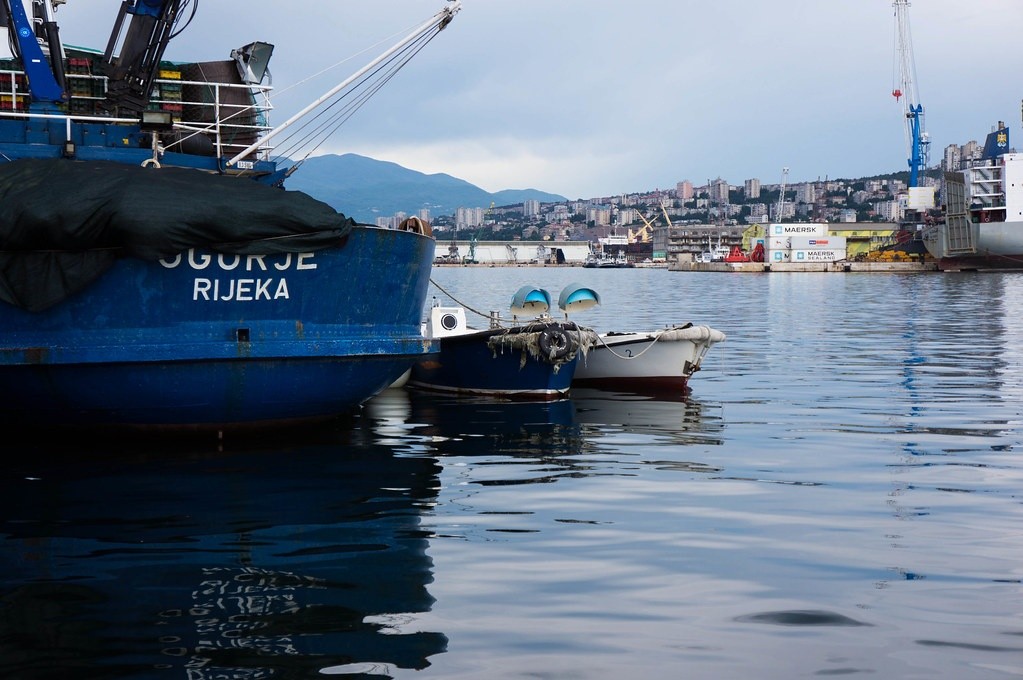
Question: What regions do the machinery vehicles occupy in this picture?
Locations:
[505,244,518,265]
[462,201,494,264]
[431,215,463,264]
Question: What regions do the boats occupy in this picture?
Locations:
[616,250,635,268]
[700,237,715,262]
[921,121,1023,261]
[582,241,599,268]
[507,284,726,393]
[0,0,465,443]
[711,234,730,260]
[597,240,617,268]
[597,220,654,263]
[408,297,597,405]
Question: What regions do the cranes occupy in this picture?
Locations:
[776,163,789,223]
[891,0,931,187]
